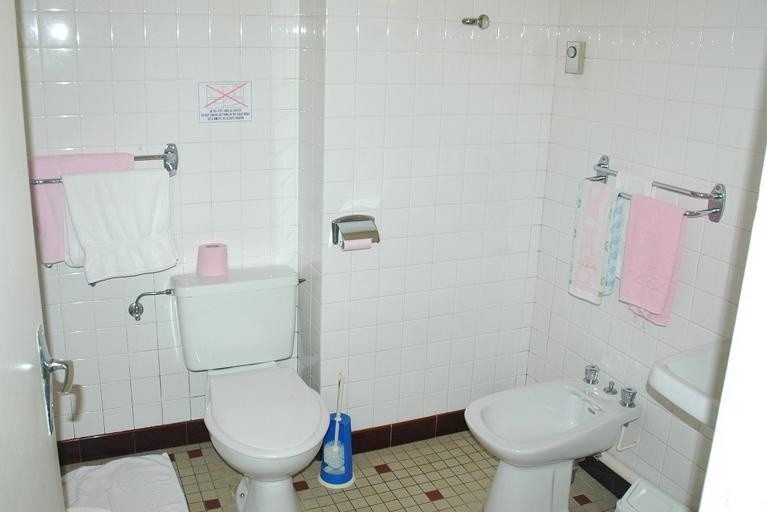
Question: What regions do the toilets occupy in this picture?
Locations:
[170,267,332,511]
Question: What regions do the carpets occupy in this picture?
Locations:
[61,452,191,512]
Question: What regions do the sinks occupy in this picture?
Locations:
[465,379,643,466]
[648,335,732,428]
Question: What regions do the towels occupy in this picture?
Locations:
[27,154,136,265]
[618,195,685,328]
[565,180,618,305]
[63,170,180,285]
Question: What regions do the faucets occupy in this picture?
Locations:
[604,380,618,395]
